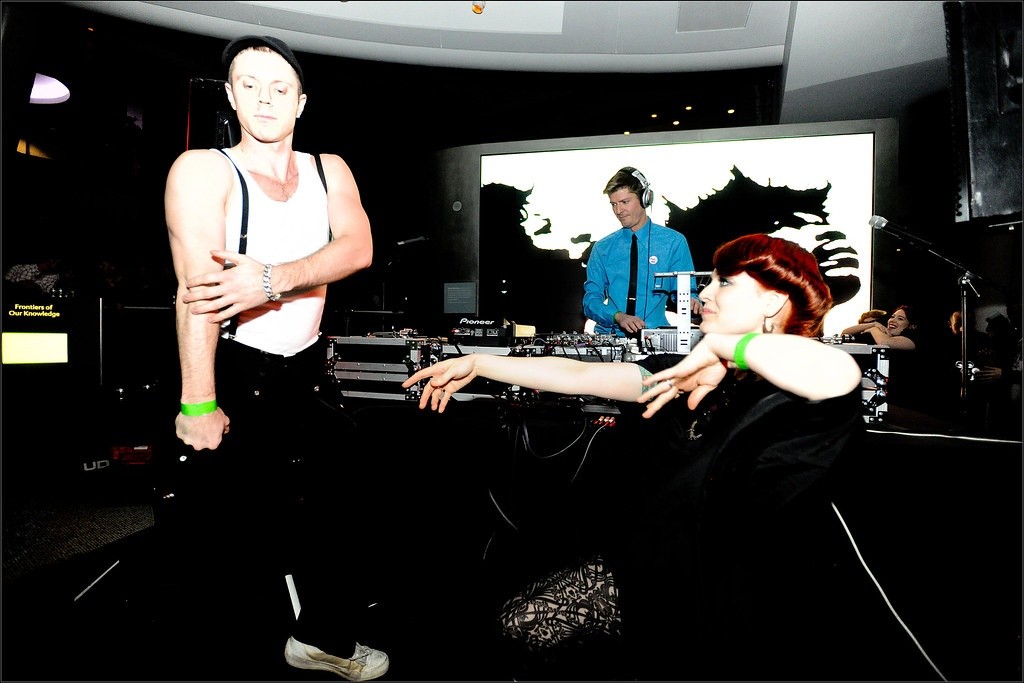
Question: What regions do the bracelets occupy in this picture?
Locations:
[734,332,760,370]
[263,263,282,302]
[613,311,621,321]
[180,399,217,416]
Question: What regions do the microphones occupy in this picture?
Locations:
[868,215,931,249]
[398,236,429,246]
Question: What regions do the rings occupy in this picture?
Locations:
[666,379,672,388]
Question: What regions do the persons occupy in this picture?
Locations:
[164,35,390,682]
[983,365,1022,385]
[402,233,863,651]
[947,310,995,366]
[584,167,703,337]
[842,305,945,419]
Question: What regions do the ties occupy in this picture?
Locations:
[625,234,638,337]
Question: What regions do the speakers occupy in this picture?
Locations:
[943,0,1024,224]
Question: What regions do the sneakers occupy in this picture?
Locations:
[283,634,391,683]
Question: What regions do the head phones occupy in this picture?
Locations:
[619,167,654,209]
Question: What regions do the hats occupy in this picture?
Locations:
[220,34,305,93]
